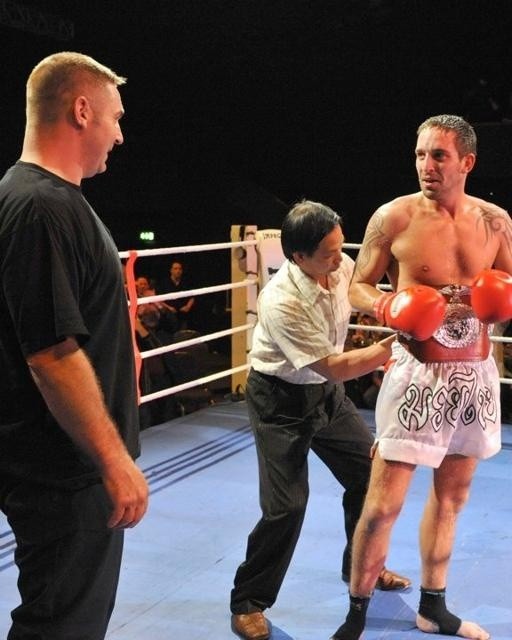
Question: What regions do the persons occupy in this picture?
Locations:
[121,259,195,431]
[0,48,152,640]
[329,113,512,640]
[343,312,395,411]
[227,196,397,640]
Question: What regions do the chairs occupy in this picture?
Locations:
[162,330,217,417]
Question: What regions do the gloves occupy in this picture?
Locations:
[373,284,445,342]
[471,269,512,324]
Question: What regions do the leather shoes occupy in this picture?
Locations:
[232,612,270,640]
[342,567,410,590]
